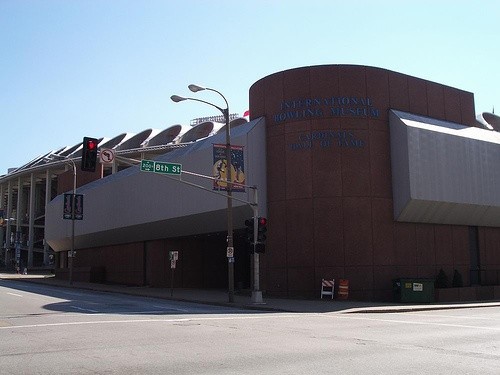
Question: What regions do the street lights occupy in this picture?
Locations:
[42,153,77,285]
[169,83,235,304]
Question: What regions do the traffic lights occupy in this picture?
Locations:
[245,218,253,241]
[80,136,98,172]
[257,216,268,242]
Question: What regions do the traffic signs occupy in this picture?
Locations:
[154,161,182,176]
[141,159,154,172]
